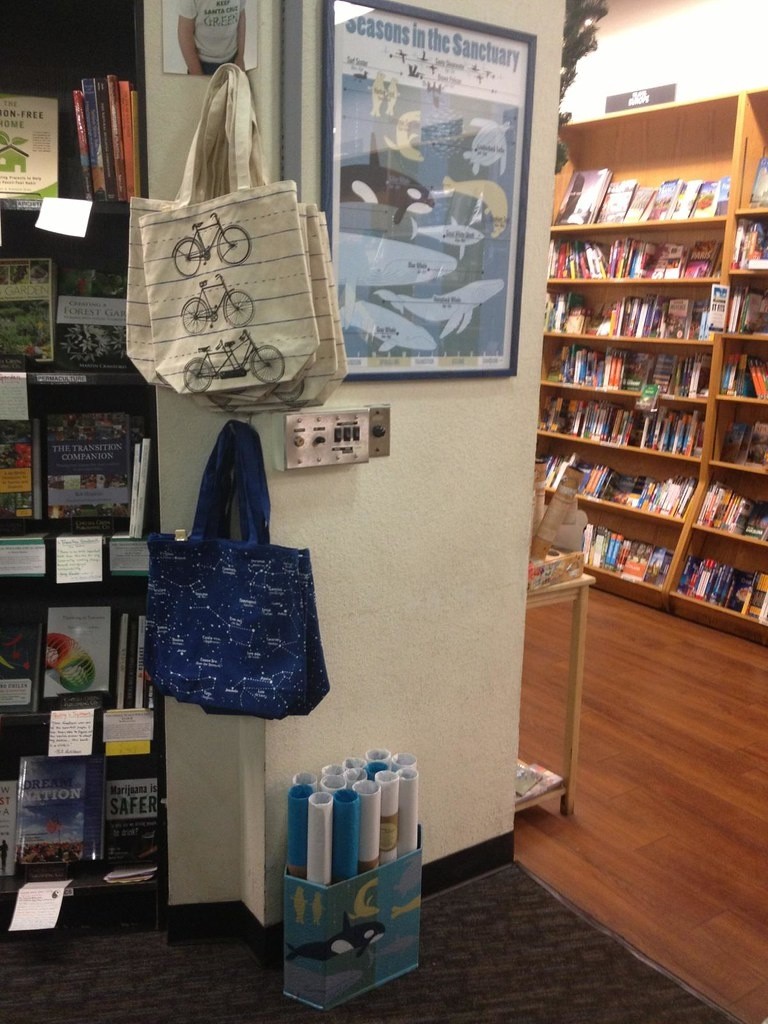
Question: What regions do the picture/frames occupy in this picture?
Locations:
[318,0,538,381]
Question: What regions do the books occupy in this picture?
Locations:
[547,345,710,398]
[552,167,727,225]
[677,555,768,624]
[730,218,768,271]
[541,290,710,345]
[707,286,766,336]
[0,75,168,912]
[538,447,698,519]
[696,479,767,542]
[548,235,723,285]
[745,147,768,209]
[721,419,768,469]
[538,393,703,459]
[578,522,674,590]
[720,353,767,399]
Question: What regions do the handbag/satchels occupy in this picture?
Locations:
[150,420,335,722]
[128,63,347,413]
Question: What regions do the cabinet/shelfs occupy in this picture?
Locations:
[666,87,768,647]
[0,0,171,934]
[532,88,747,615]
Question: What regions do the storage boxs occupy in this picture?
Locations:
[283,823,422,1009]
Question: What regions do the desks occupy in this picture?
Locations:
[514,572,598,818]
